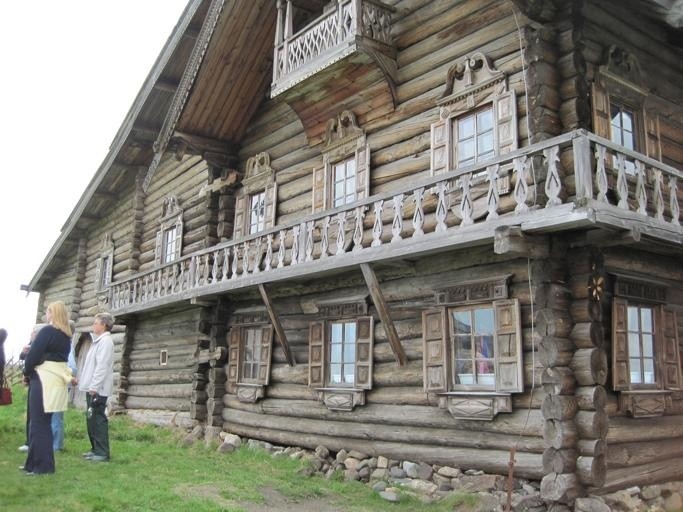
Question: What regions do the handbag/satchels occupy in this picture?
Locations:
[0,378,12,406]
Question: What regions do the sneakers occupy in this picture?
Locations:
[18,445,29,451]
[83,452,110,462]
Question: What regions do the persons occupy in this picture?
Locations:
[18,300,115,476]
[0,328,12,405]
